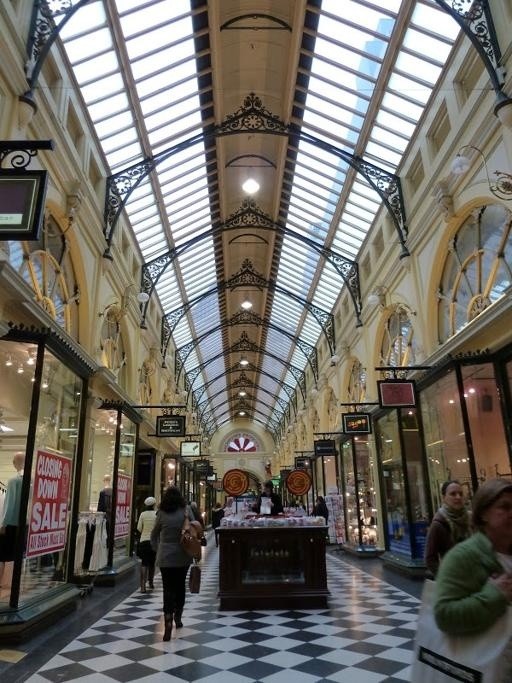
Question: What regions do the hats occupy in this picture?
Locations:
[143,496,156,506]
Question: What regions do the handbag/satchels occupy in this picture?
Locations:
[201,536,206,545]
[189,566,201,593]
[180,504,204,563]
[409,575,512,681]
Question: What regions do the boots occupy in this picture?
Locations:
[140,565,147,592]
[147,567,154,587]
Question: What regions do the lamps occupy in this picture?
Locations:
[295,284,419,417]
[173,390,209,443]
[98,281,150,324]
[448,141,511,201]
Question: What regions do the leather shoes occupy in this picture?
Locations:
[175,621,183,629]
[163,621,172,641]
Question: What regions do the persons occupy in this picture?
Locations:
[212,502,225,546]
[298,499,307,509]
[137,484,204,642]
[1,452,26,594]
[98,474,111,512]
[253,480,283,516]
[432,479,512,635]
[426,480,473,575]
[312,496,328,525]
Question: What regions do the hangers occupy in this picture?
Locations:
[75,510,105,527]
